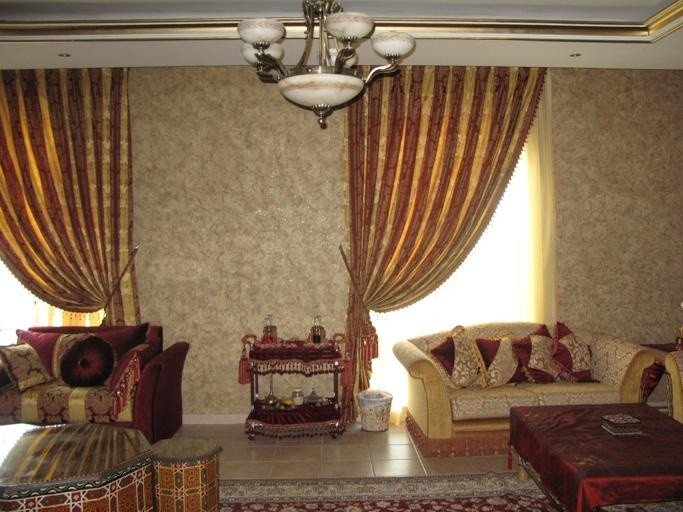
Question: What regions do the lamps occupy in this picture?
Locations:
[236,0,419,125]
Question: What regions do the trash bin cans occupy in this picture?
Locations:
[357,389,393,432]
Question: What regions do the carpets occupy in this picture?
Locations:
[217,476,554,511]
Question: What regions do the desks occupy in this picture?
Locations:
[0,423,223,510]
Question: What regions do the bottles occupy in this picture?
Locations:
[310,317,324,344]
[256,375,329,410]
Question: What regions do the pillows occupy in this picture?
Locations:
[428,323,595,387]
[0,331,152,391]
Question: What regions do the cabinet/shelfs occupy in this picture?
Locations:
[246,338,347,439]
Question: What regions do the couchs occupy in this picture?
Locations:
[392,324,670,439]
[0,325,189,441]
